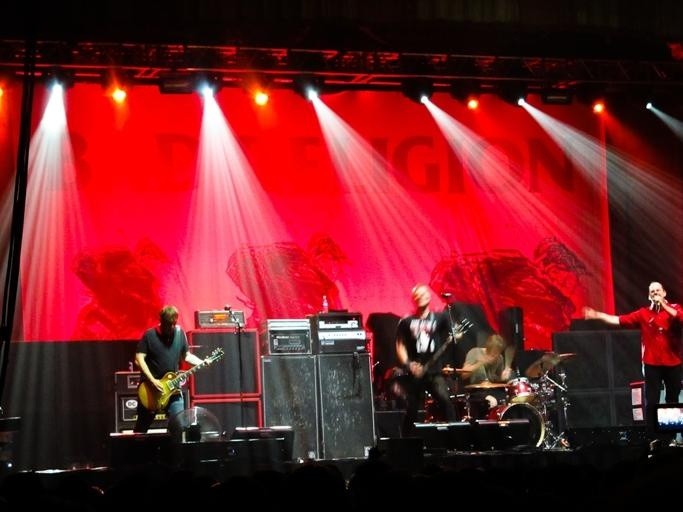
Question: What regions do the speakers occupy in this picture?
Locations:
[261,355,320,460]
[190,398,264,436]
[317,353,377,460]
[234,426,294,459]
[179,329,262,399]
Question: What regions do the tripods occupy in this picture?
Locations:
[541,374,567,450]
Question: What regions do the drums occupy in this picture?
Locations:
[489,403,545,448]
[506,377,535,403]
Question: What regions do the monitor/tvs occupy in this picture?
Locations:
[654,404,683,432]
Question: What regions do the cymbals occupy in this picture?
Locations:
[442,367,472,374]
[526,359,551,379]
[465,380,511,389]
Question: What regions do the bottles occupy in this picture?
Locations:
[675,432,683,447]
[322,295,329,313]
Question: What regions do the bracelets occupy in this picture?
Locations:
[405,360,412,369]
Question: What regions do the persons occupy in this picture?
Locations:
[586,281,683,426]
[133,304,211,442]
[392,284,464,422]
[1,440,683,510]
[462,334,507,417]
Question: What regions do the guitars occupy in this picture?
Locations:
[138,348,224,412]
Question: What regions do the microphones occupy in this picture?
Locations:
[442,293,452,297]
[656,301,661,313]
[224,305,232,310]
